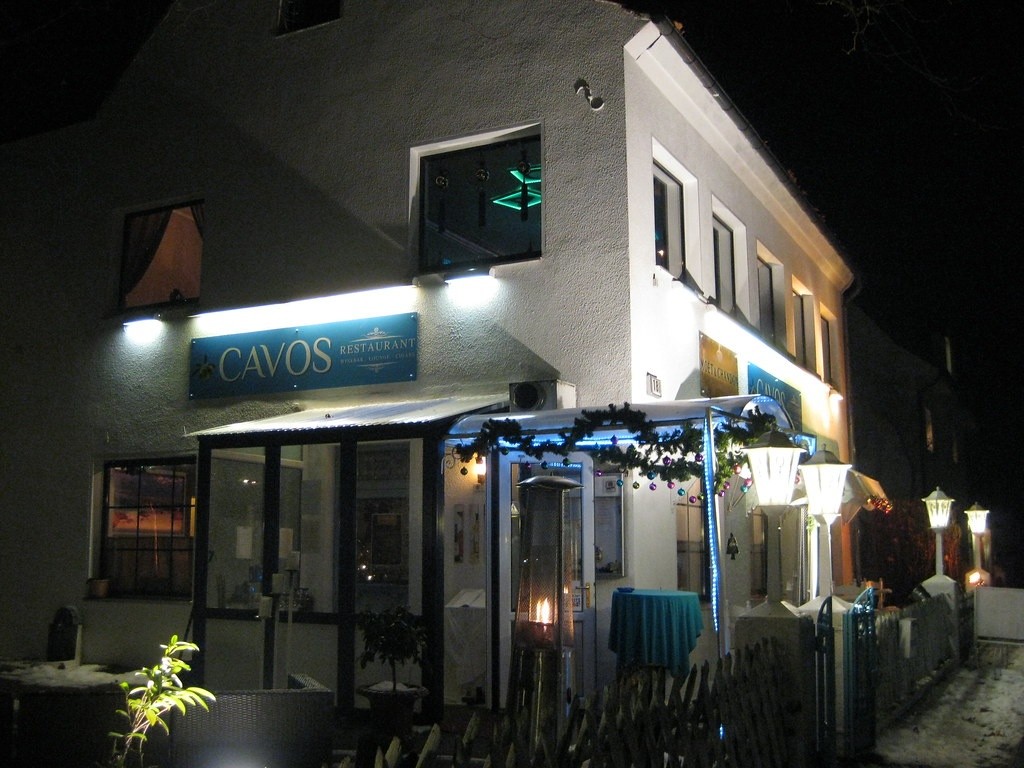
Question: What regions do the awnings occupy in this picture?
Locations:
[731,449,889,528]
[446,392,797,448]
[181,394,512,705]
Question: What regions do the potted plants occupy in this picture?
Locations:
[349,603,438,733]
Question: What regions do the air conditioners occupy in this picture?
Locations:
[507,379,578,415]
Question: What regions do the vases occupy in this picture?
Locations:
[88,577,112,598]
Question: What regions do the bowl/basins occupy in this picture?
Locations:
[139,575,174,601]
[89,580,113,599]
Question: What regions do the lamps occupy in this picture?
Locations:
[726,533,741,561]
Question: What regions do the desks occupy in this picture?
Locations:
[608,587,705,680]
